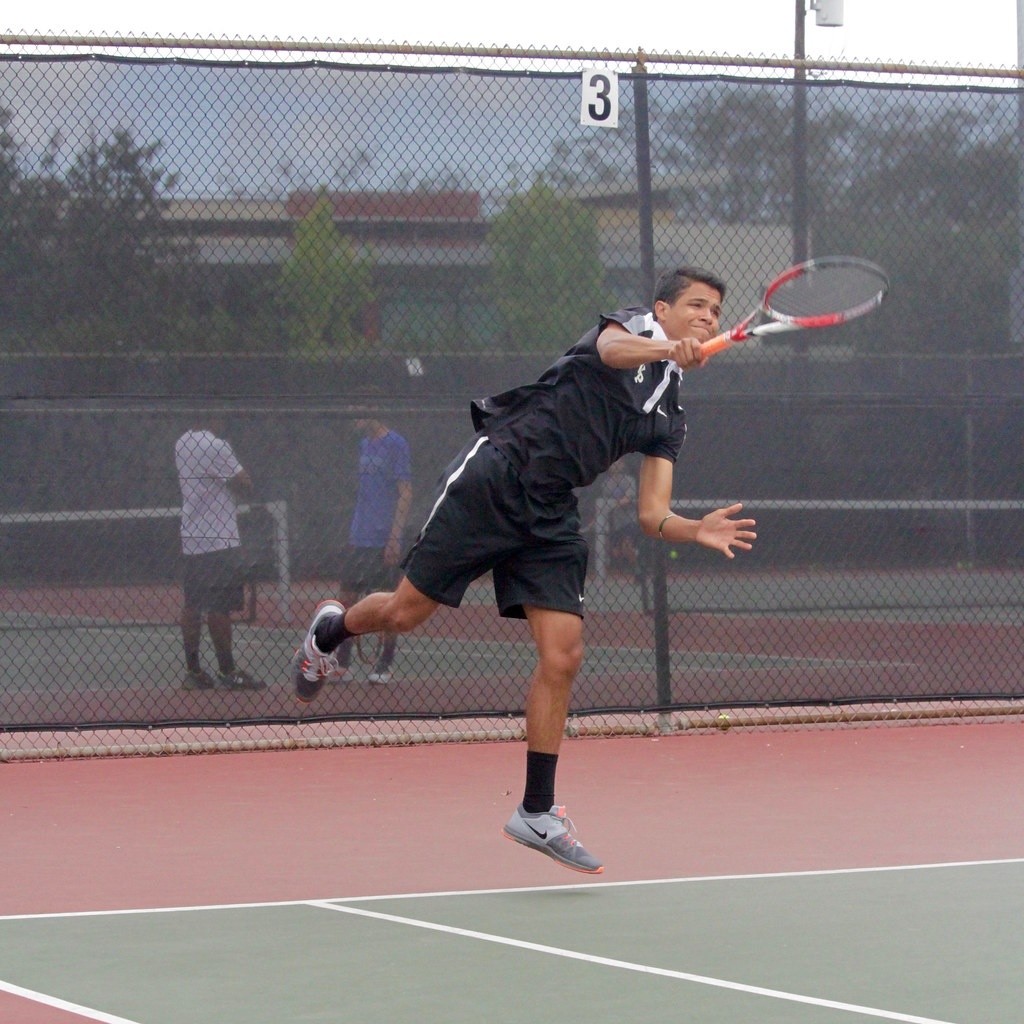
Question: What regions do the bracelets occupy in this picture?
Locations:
[657,513,679,541]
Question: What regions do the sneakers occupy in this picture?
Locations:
[370,662,396,685]
[183,670,215,690]
[229,672,268,689]
[290,600,347,705]
[499,803,605,875]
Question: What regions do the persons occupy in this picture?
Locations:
[174,393,263,692]
[287,265,756,875]
[580,455,646,586]
[329,382,414,683]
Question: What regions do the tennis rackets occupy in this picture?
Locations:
[701,259,892,357]
